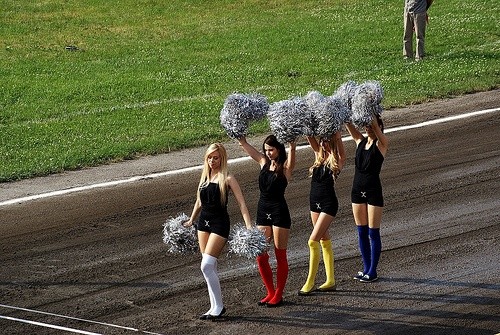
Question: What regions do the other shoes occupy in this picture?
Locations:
[360,275,378,282]
[353,272,364,279]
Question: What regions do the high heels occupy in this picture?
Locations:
[209,306,226,322]
[265,300,283,307]
[316,283,336,292]
[298,286,316,296]
[258,297,269,305]
[201,314,209,319]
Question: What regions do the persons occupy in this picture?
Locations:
[343,112,389,283]
[298,130,346,296]
[235,134,296,308]
[182,143,254,321]
[402,0,433,62]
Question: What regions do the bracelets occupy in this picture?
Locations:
[239,139,246,146]
[289,148,295,151]
